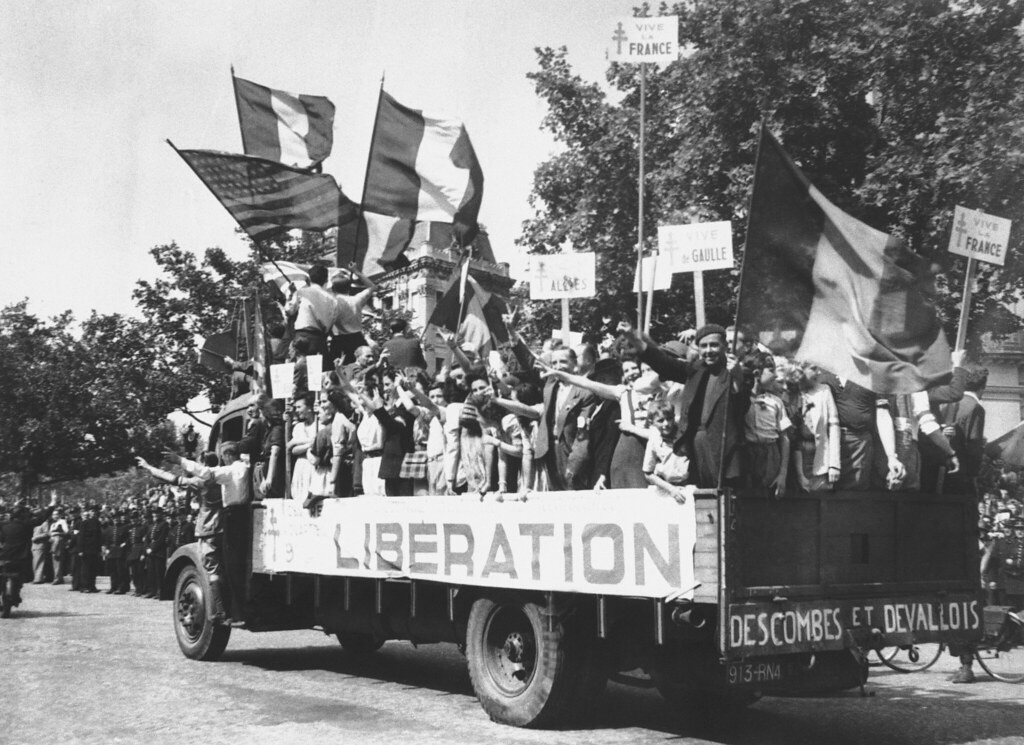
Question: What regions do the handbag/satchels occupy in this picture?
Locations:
[399,452,426,479]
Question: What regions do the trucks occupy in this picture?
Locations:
[160,392,984,728]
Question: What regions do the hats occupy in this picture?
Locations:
[152,507,165,514]
[130,511,139,519]
[586,358,623,381]
[174,508,186,516]
[696,324,725,344]
[68,507,80,514]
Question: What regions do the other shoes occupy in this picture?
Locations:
[80,587,91,593]
[144,592,156,598]
[135,592,144,597]
[115,587,126,594]
[106,588,115,594]
[52,578,63,585]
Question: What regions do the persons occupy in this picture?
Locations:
[47,484,201,598]
[280,267,984,501]
[943,457,1024,683]
[130,356,292,627]
[0,488,58,618]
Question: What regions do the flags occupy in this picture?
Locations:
[180,148,361,241]
[234,78,337,171]
[334,197,415,278]
[261,260,358,301]
[737,123,954,396]
[423,277,511,363]
[363,92,485,230]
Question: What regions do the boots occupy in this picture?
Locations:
[210,580,227,621]
